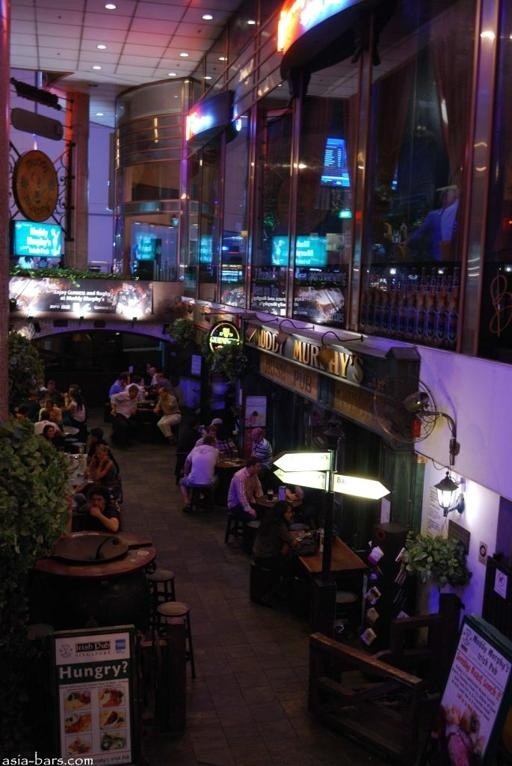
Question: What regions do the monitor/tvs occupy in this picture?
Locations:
[319,136,399,191]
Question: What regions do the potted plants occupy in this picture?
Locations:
[403,530,473,588]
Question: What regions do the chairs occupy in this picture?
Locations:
[16,389,123,531]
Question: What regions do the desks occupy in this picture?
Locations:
[32,532,156,627]
[135,392,162,447]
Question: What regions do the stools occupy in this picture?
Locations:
[170,423,336,611]
[152,600,195,680]
[143,568,177,640]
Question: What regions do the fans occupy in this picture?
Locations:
[373,370,460,465]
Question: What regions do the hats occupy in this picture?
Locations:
[210,418,223,426]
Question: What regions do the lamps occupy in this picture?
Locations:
[317,330,363,371]
[434,471,464,517]
[241,314,278,342]
[276,318,314,345]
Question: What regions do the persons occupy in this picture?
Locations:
[397,184,463,263]
[420,703,452,766]
[18,257,61,270]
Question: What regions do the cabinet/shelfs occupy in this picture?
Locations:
[362,523,421,654]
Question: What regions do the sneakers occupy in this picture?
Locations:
[182,501,193,512]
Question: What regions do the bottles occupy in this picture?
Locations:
[77,446,86,474]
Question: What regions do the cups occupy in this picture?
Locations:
[232,449,239,460]
[267,490,275,500]
